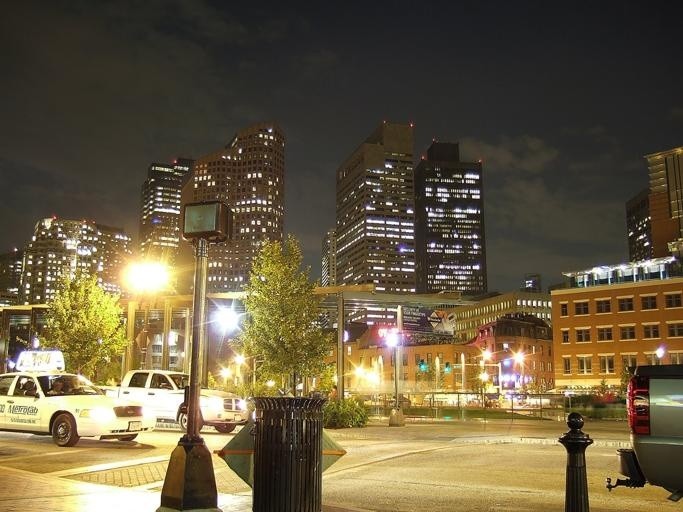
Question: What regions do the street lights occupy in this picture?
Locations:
[654,347,663,366]
[385,333,398,407]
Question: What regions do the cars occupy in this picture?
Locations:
[0,371,157,447]
[363,393,411,409]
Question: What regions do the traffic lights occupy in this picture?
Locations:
[445,361,450,373]
[418,359,424,369]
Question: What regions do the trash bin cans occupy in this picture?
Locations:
[252,398,328,511]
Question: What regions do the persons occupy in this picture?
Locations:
[169,376,179,388]
[326,385,337,400]
[45,378,65,395]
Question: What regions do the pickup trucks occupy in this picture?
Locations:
[606,364,682,503]
[96,369,249,433]
